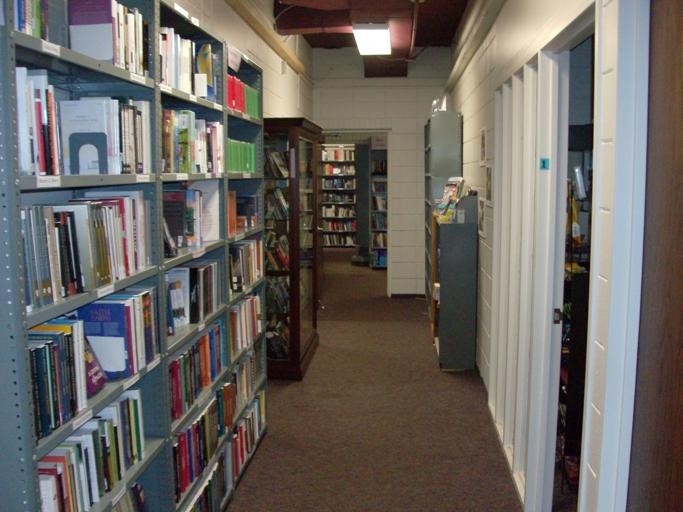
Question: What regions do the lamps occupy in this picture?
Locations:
[351,19,393,57]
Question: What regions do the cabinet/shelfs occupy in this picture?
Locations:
[430,195,482,375]
[263,116,326,382]
[226,47,267,511]
[424,112,464,317]
[323,135,388,268]
[158,1,229,511]
[0,0,158,512]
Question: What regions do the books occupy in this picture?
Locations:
[372,158,388,265]
[321,146,356,248]
[14,0,266,511]
[260,148,313,359]
[437,176,473,214]
